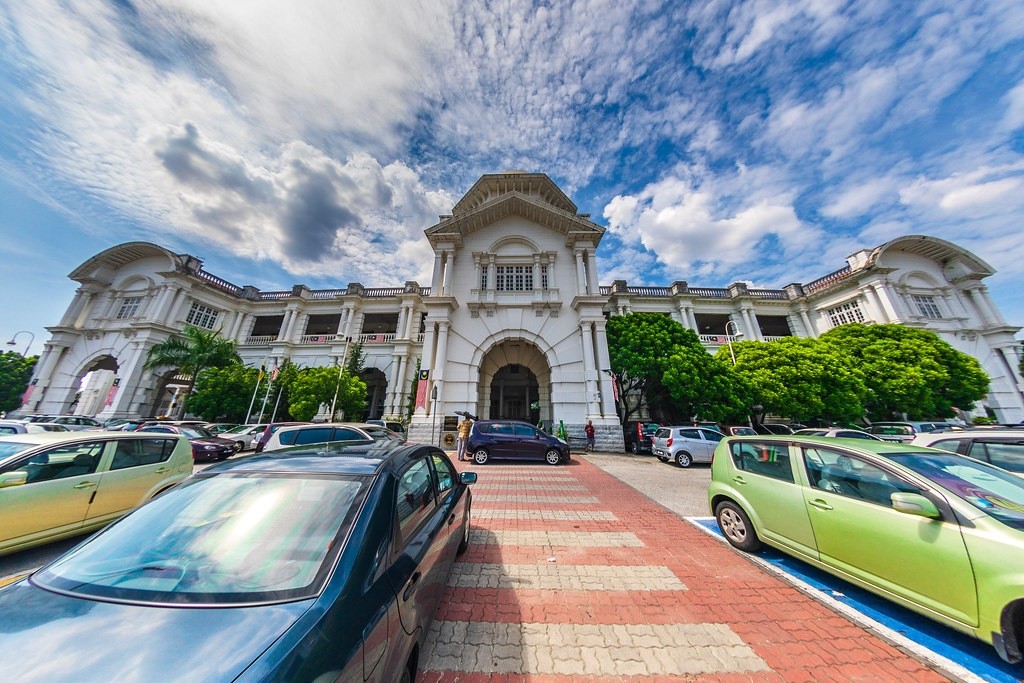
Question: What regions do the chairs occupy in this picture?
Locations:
[324,482,361,533]
[53,454,94,479]
[857,463,902,506]
[14,451,50,483]
[818,464,864,498]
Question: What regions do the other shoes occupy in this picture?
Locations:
[585,448,588,451]
[458,458,467,461]
[592,450,594,452]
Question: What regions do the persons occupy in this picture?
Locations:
[585,420,595,452]
[456,415,473,461]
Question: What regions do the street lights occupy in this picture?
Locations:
[725,321,753,428]
[330,321,351,418]
[7,331,35,357]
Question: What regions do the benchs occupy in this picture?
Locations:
[739,451,817,489]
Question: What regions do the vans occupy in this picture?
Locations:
[365,420,408,439]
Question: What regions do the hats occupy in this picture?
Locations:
[588,420,592,424]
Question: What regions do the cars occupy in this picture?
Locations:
[454,411,570,464]
[652,427,728,469]
[695,419,1024,481]
[709,434,1024,666]
[0,414,404,464]
[0,430,193,558]
[1,440,477,683]
[911,430,1024,479]
[623,420,665,455]
